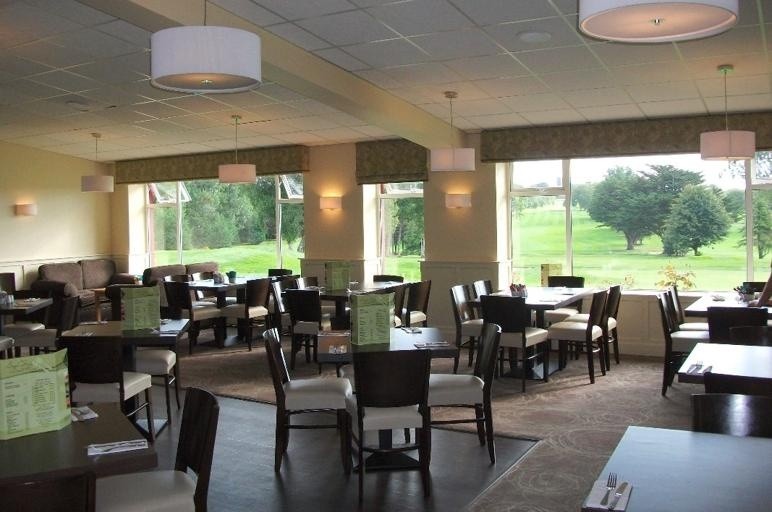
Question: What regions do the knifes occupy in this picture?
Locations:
[607,483,628,508]
[81,439,147,448]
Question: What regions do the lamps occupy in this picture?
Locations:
[698,65,757,162]
[150,0,263,95]
[577,1,740,46]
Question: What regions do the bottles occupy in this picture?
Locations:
[214,271,237,283]
[7,293,14,304]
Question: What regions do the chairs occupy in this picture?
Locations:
[654,278,772,440]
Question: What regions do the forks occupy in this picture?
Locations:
[599,472,617,505]
[688,360,702,373]
